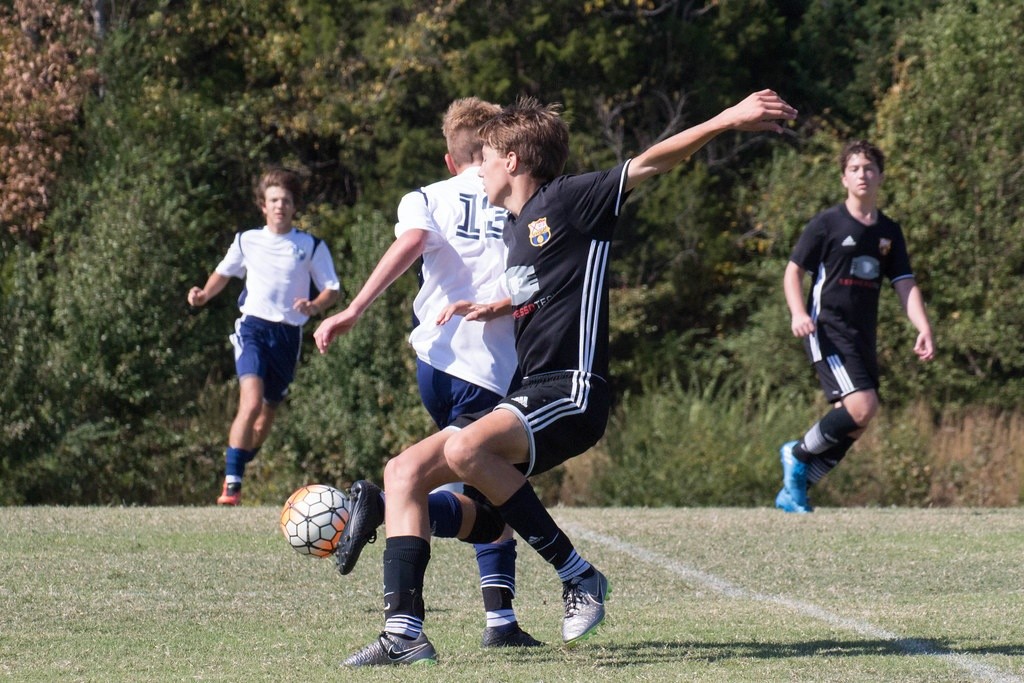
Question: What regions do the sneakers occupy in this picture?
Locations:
[338,633,438,666]
[334,481,385,576]
[776,485,813,511]
[781,443,807,506]
[562,566,610,643]
[480,623,548,647]
[216,480,241,505]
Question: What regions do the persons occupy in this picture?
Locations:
[777,140,937,516]
[186,170,339,508]
[345,88,799,668]
[314,96,547,650]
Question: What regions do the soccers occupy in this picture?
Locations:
[279,483,349,559]
